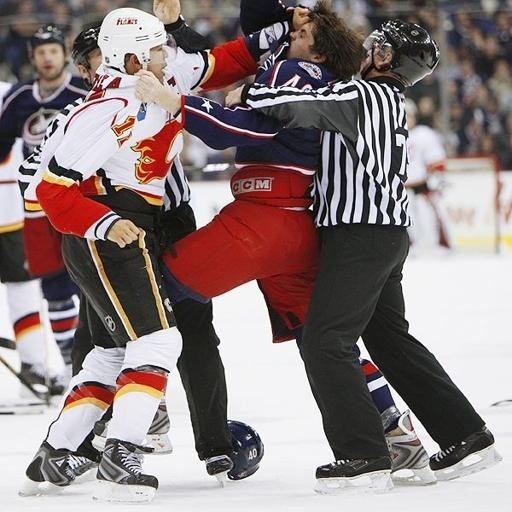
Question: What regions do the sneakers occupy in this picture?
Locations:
[68,448,98,476]
[93,400,171,439]
[26,438,76,488]
[429,425,494,472]
[381,409,428,470]
[202,453,232,475]
[97,437,159,491]
[315,456,391,480]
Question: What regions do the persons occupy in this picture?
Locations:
[225,20,503,495]
[0,82,64,405]
[17,7,310,503]
[405,98,451,249]
[91,0,436,488]
[0,25,90,378]
[18,0,233,476]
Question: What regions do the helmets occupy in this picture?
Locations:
[378,19,441,87]
[228,420,265,480]
[98,8,168,69]
[30,25,66,49]
[72,26,101,65]
